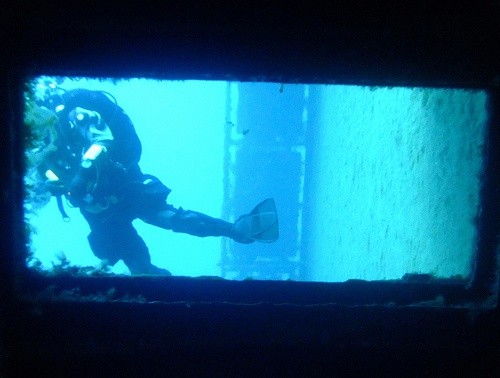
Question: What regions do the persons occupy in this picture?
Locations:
[34,88,255,279]
